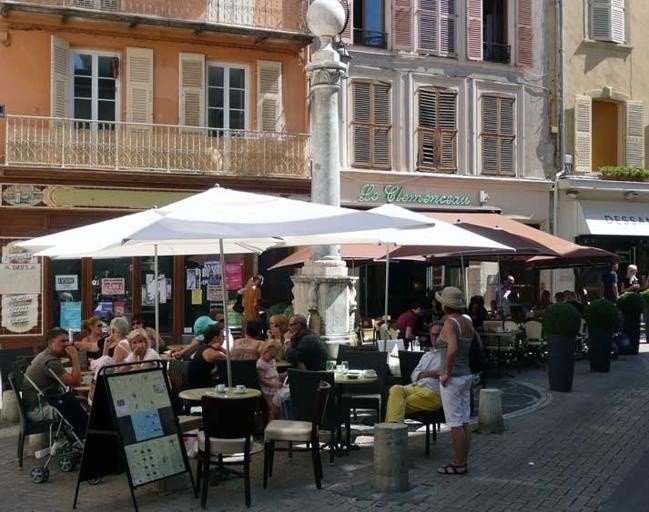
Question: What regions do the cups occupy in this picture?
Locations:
[216,384,225,390]
[326,360,377,379]
[236,384,245,391]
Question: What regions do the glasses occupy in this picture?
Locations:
[131,320,143,326]
[91,322,104,328]
[287,321,300,328]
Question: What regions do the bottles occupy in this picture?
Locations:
[414,336,422,351]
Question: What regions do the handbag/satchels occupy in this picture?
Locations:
[232,293,245,314]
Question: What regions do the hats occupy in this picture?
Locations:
[434,286,467,310]
[193,315,219,334]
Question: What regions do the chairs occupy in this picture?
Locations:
[10,298,624,511]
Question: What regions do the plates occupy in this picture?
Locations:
[216,390,228,393]
[233,391,247,394]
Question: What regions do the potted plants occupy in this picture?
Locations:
[616,291,642,354]
[639,288,649,344]
[598,164,649,183]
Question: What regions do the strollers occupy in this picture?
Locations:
[18,362,103,486]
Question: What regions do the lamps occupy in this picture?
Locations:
[624,191,638,200]
[567,190,579,198]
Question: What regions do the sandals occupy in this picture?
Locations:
[437,460,468,475]
